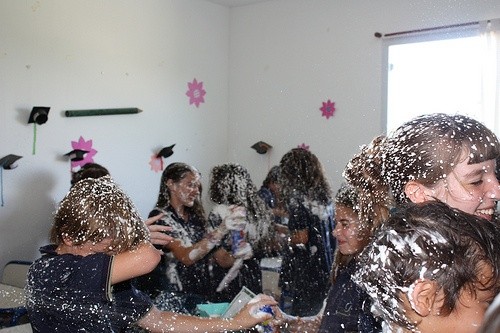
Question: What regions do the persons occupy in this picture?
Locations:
[24,113,500,333]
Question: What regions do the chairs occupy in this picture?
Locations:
[0,260,32,327]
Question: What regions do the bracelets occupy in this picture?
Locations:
[287,235,292,247]
[206,232,221,246]
